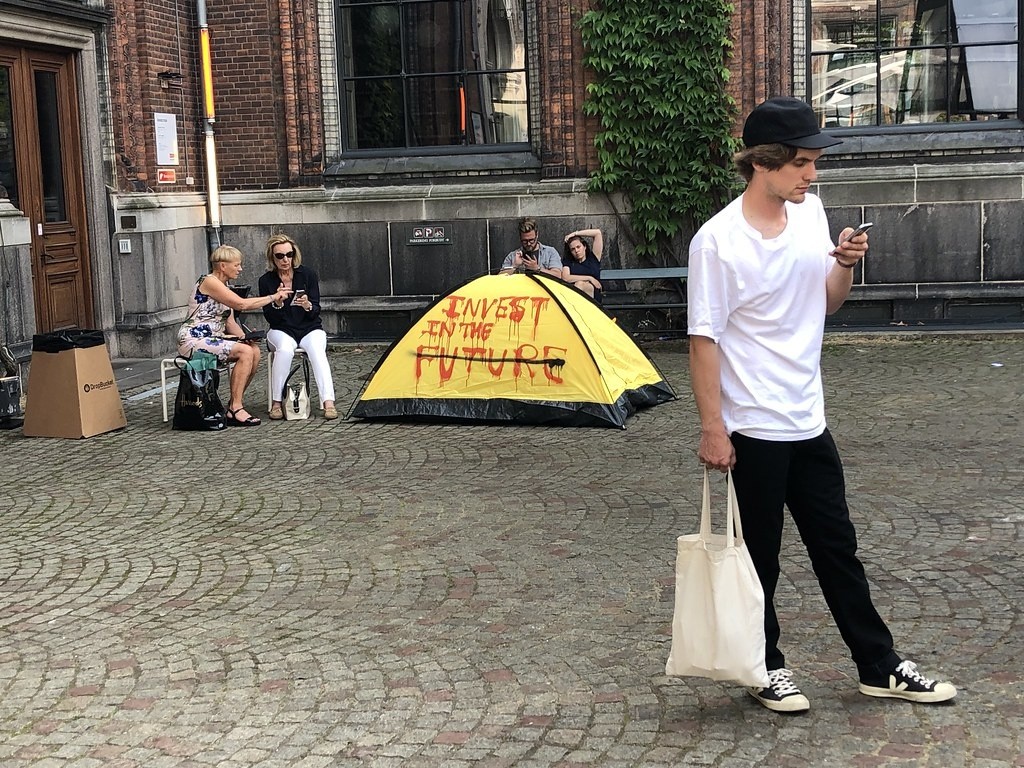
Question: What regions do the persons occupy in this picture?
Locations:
[686,95,957,712]
[175,244,294,427]
[499,221,563,279]
[560,228,603,303]
[258,234,338,419]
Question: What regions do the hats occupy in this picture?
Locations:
[742,97,844,149]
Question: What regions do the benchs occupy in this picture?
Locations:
[599,267,689,333]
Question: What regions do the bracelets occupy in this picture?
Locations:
[576,231,579,236]
[836,257,859,268]
[269,295,276,301]
[536,266,542,271]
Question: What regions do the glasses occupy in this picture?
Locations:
[520,232,537,243]
[272,251,293,260]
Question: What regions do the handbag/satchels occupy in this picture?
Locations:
[172,348,228,431]
[283,358,311,420]
[664,460,770,689]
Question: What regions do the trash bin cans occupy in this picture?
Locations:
[22,327,128,439]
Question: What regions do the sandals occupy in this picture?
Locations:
[226,407,261,426]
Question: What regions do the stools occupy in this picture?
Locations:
[161,348,324,422]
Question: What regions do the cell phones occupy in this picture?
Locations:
[240,330,264,341]
[290,289,305,306]
[831,222,873,258]
[523,251,532,266]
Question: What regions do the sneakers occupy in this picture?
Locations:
[857,659,957,703]
[744,669,810,711]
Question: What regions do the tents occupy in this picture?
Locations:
[341,266,681,431]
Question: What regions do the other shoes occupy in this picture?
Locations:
[324,407,338,419]
[269,406,283,419]
